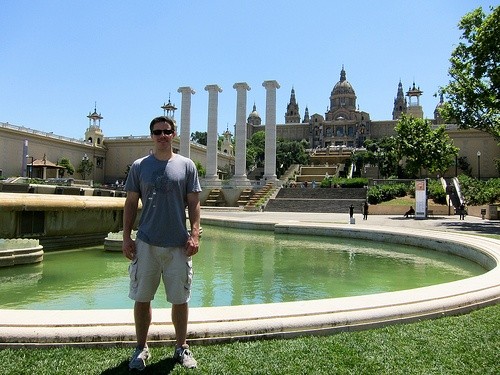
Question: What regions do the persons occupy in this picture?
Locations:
[404,206,415,218]
[459,203,465,220]
[363,201,369,220]
[122,117,202,370]
[348,204,354,219]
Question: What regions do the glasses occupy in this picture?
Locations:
[152,130,174,136]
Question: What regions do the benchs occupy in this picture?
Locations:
[427,210,433,218]
[410,211,415,218]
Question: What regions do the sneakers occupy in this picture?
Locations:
[129,344,151,369]
[174,345,197,368]
